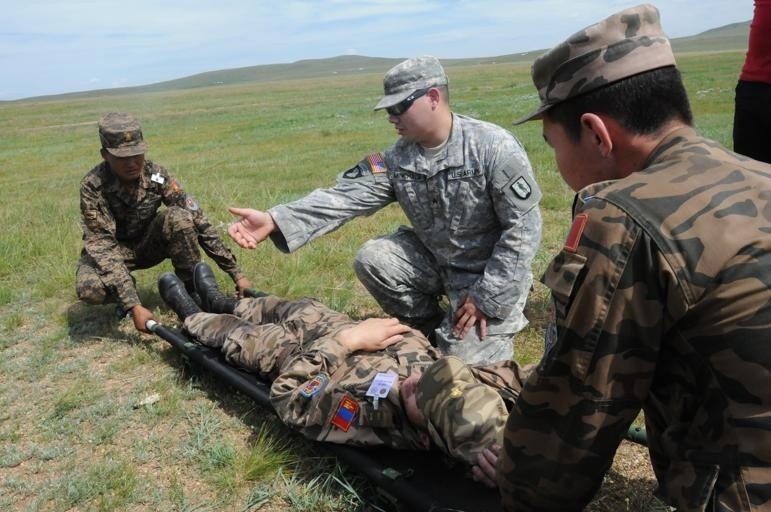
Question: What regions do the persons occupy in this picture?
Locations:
[732,0,771,164]
[473,3,771,511]
[72,110,255,335]
[224,53,541,364]
[154,259,536,488]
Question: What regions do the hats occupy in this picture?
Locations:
[371,54,449,112]
[415,355,511,466]
[509,3,678,127]
[98,110,148,159]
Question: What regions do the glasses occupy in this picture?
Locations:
[384,87,431,116]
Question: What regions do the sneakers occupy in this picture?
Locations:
[113,273,136,322]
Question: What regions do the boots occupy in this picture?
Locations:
[192,261,240,314]
[157,271,205,323]
